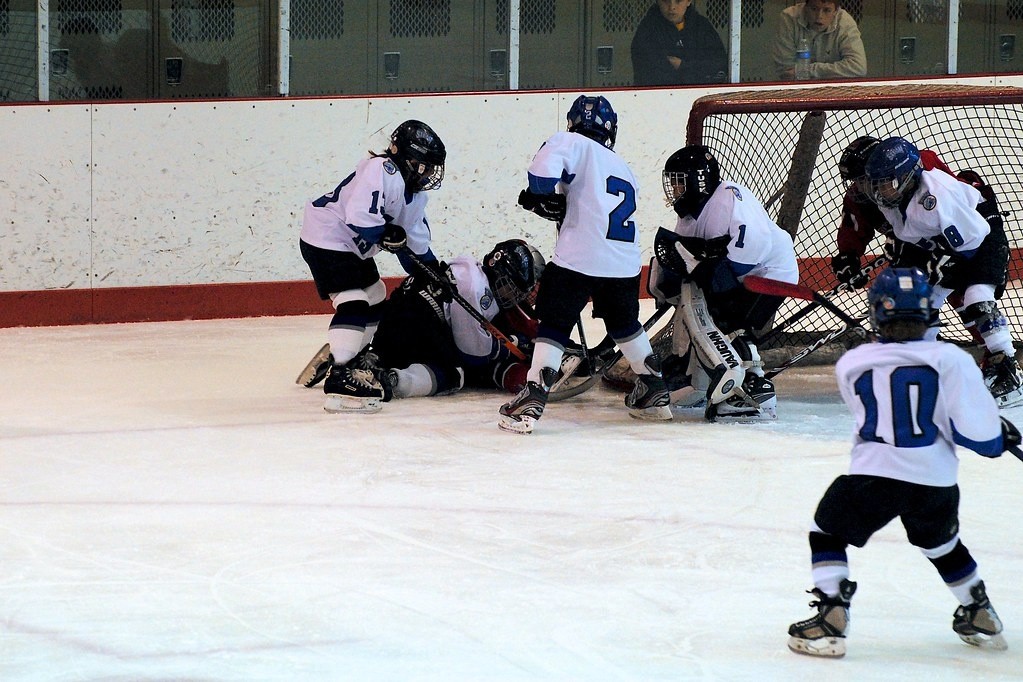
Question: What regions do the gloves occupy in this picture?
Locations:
[974,234,1011,286]
[833,252,870,293]
[1000,416,1021,454]
[377,223,407,253]
[422,261,458,303]
[521,187,566,222]
[491,333,531,364]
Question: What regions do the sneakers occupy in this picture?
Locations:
[624,375,673,422]
[714,373,777,423]
[953,597,1008,651]
[499,382,546,434]
[322,353,392,415]
[789,597,846,657]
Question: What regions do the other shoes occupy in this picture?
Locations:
[982,355,1023,409]
[296,343,333,387]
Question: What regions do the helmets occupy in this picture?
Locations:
[869,266,930,325]
[567,95,618,150]
[386,120,447,191]
[836,136,882,202]
[865,137,924,210]
[662,145,720,218]
[481,239,546,310]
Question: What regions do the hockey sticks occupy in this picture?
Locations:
[565,334,616,356]
[743,274,1023,462]
[545,177,792,402]
[764,310,870,380]
[555,220,595,377]
[400,244,532,364]
[758,253,888,346]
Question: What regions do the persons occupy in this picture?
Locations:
[833,135,1023,410]
[773,0,868,80]
[784,265,1022,658]
[297,120,456,412]
[296,93,799,433]
[631,0,728,86]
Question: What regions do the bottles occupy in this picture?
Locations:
[795,38,811,80]
